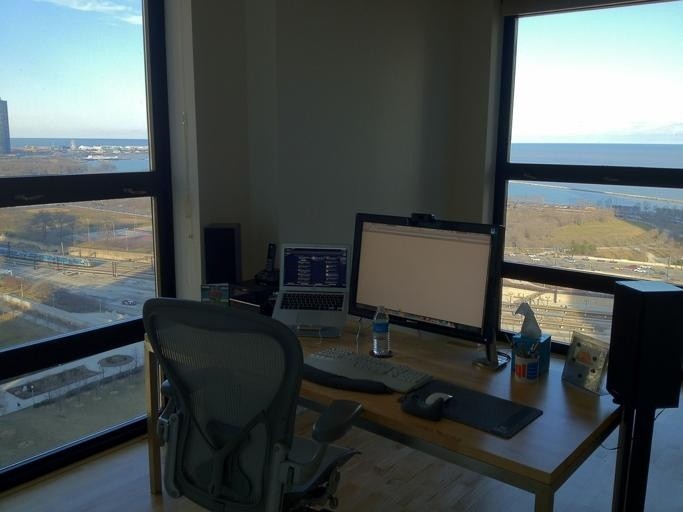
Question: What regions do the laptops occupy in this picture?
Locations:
[271,244,351,329]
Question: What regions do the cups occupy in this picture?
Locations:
[514,352,540,384]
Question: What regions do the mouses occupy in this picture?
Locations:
[418,392,454,409]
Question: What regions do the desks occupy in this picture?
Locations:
[143,314,635,512]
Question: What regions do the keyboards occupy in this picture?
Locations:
[303,345,432,396]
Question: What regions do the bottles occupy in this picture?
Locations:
[371,305,392,356]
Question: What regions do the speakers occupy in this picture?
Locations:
[202,221,242,289]
[605,277,682,408]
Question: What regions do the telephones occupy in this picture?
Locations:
[255,243,279,287]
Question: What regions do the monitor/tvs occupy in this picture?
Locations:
[348,211,505,341]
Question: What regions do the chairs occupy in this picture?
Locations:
[143,300,362,512]
